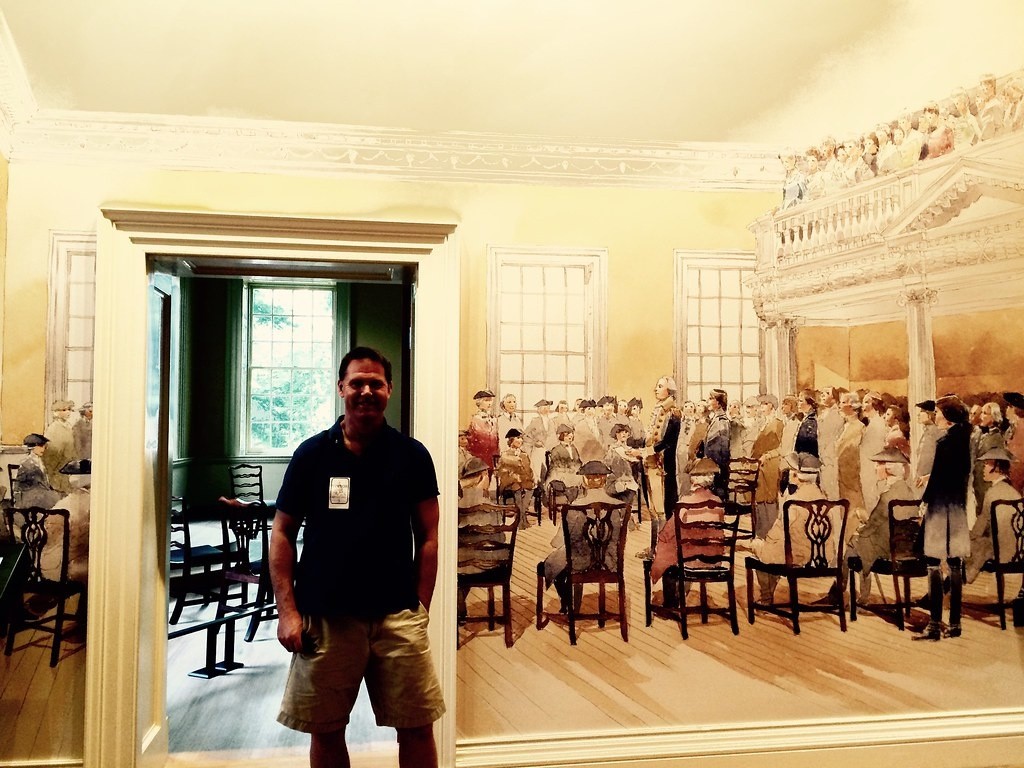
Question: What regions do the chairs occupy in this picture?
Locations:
[170,471,306,642]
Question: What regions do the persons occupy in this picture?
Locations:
[269,347,447,768]
